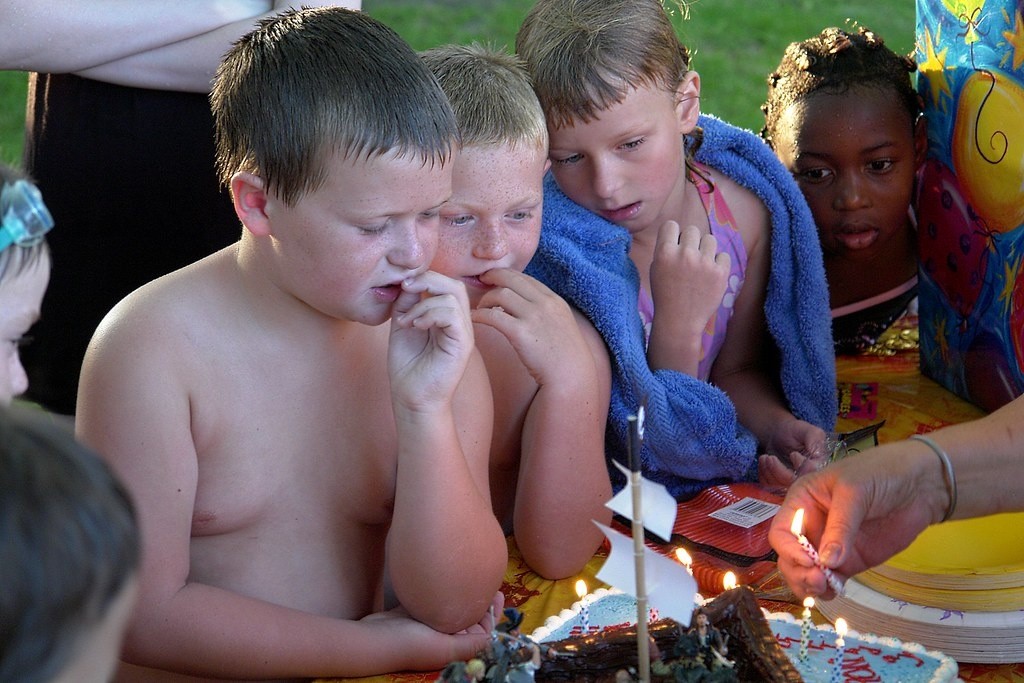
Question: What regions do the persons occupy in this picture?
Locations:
[0,0,366,422]
[66,5,510,681]
[414,33,611,582]
[514,0,828,495]
[0,400,140,682]
[756,28,920,360]
[0,159,55,416]
[766,390,1024,601]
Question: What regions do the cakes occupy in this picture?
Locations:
[436,582,967,682]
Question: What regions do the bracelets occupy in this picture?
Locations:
[909,432,960,521]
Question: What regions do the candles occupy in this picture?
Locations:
[675,548,693,575]
[830,617,848,683]
[791,508,843,592]
[575,579,589,635]
[800,598,815,665]
